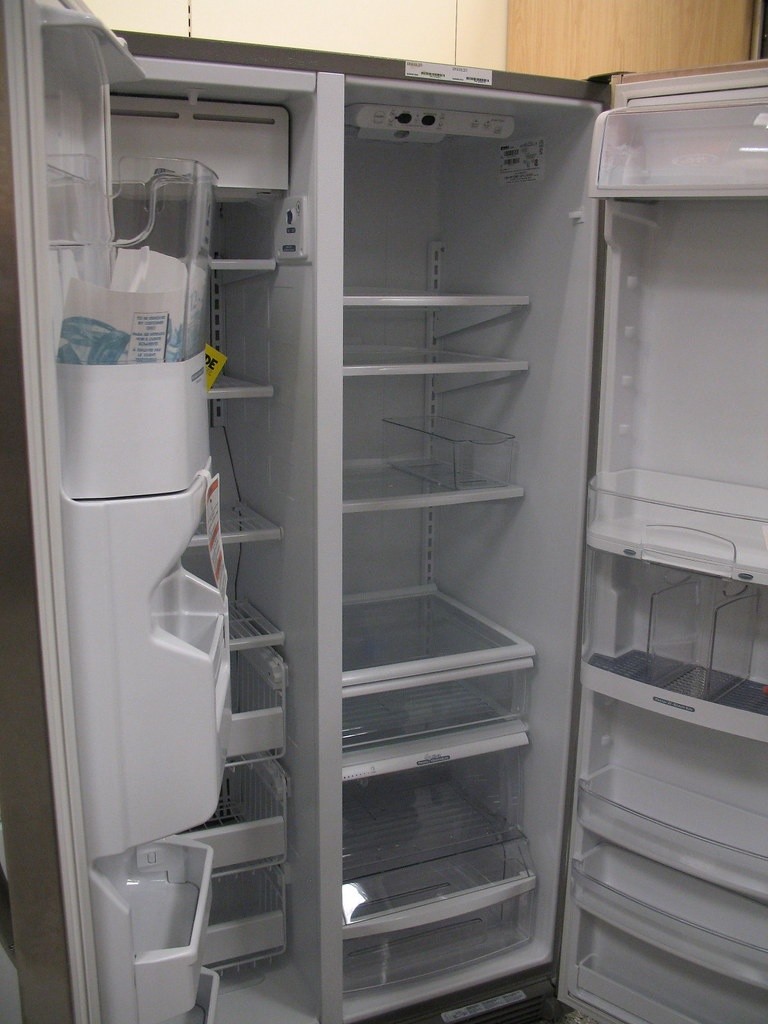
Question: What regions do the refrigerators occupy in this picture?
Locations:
[0,0,768,1024]
